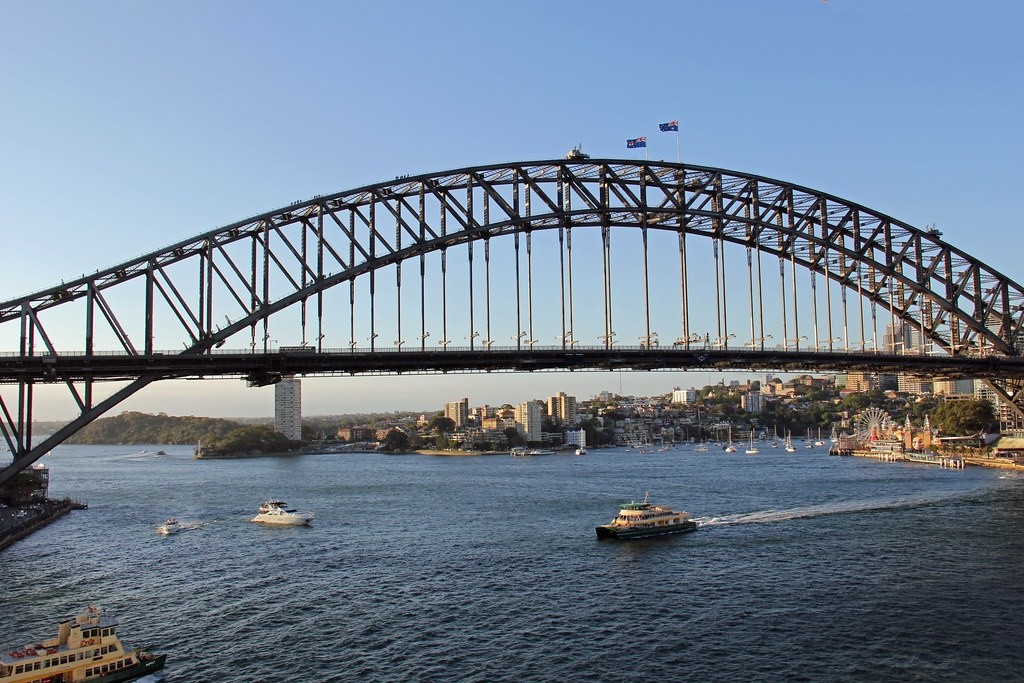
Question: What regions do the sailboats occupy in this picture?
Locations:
[771,423,797,452]
[815,426,826,446]
[725,427,737,452]
[805,427,815,449]
[745,430,760,454]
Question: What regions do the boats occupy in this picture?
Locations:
[156,450,166,455]
[529,448,557,456]
[162,518,179,534]
[140,449,147,453]
[0,607,167,683]
[594,500,697,538]
[596,434,722,454]
[259,501,297,513]
[250,504,314,524]
[575,448,587,455]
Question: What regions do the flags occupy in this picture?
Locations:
[627,137,646,148]
[659,121,677,131]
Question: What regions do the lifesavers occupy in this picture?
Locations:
[81,640,87,647]
[88,638,94,645]
